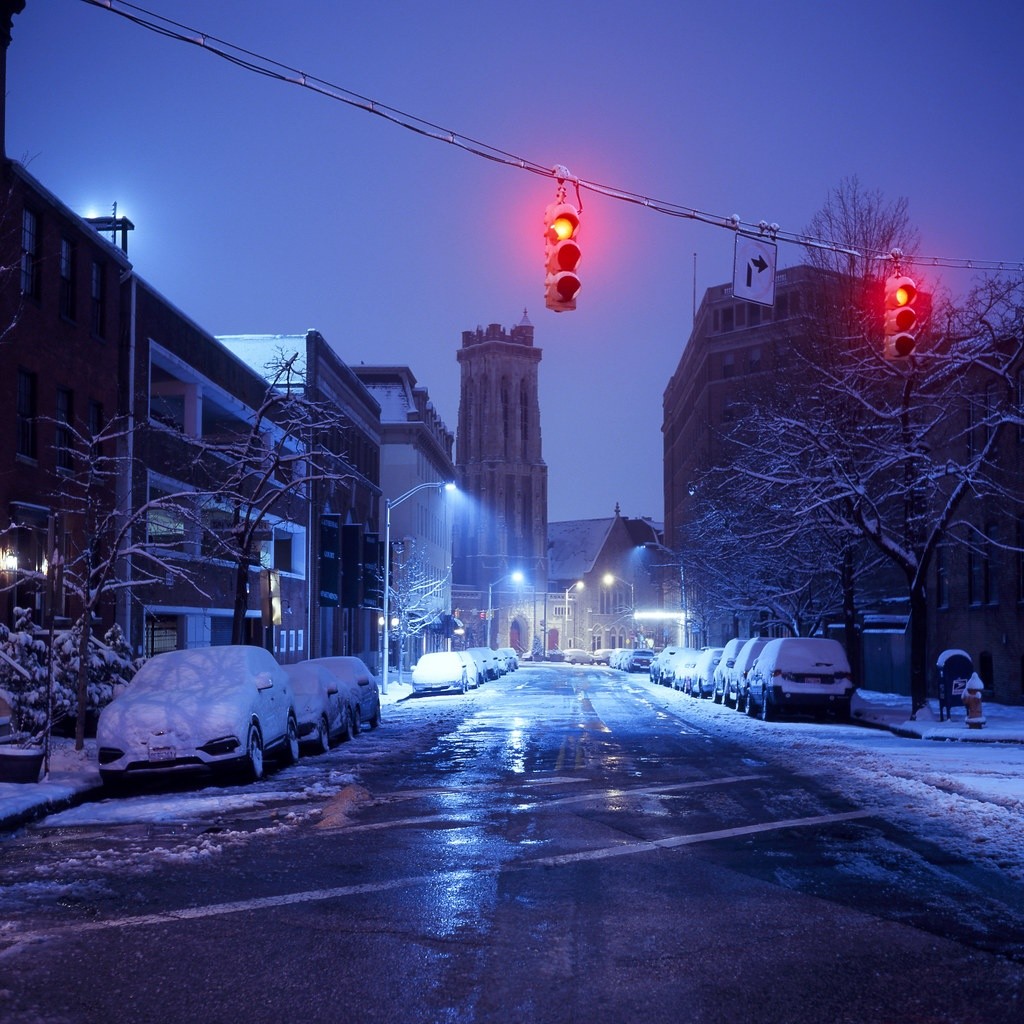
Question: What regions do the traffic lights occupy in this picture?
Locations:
[884,276,919,364]
[481,612,484,619]
[542,202,582,314]
[455,609,460,618]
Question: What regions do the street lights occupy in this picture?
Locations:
[381,479,457,696]
[603,573,634,649]
[486,571,523,648]
[564,581,585,647]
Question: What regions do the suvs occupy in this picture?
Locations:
[712,637,854,723]
[96,644,300,782]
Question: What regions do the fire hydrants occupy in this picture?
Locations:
[960,671,988,729]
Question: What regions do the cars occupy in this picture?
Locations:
[410,647,519,695]
[593,649,614,666]
[647,645,724,699]
[562,649,595,664]
[279,656,382,753]
[521,649,550,661]
[610,648,655,673]
